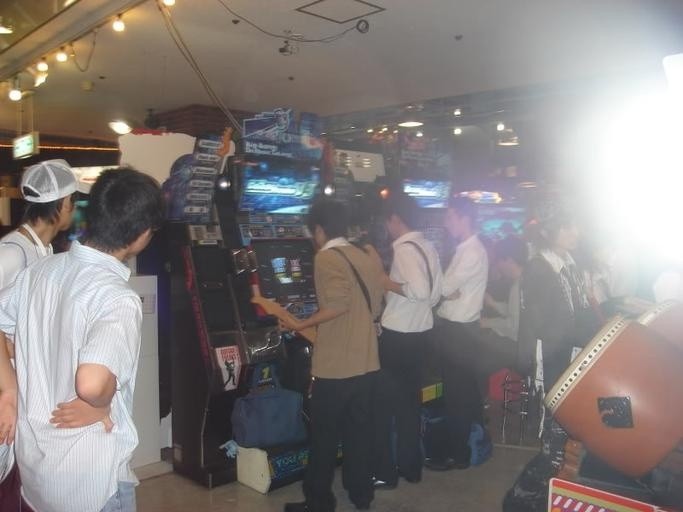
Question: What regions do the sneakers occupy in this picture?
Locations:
[424,445,492,472]
[400,469,422,483]
[349,491,370,509]
[367,472,399,490]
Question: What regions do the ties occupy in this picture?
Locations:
[564,268,581,332]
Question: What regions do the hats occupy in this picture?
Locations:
[19,158,96,204]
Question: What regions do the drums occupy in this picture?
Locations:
[637,300,682,349]
[544,313,682,480]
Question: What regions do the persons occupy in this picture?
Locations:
[0,166,161,512]
[280,200,682,510]
[0,159,94,492]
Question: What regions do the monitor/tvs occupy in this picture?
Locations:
[252,238,316,302]
[66,199,91,241]
[477,216,524,244]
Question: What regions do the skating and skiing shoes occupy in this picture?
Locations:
[283,499,336,512]
